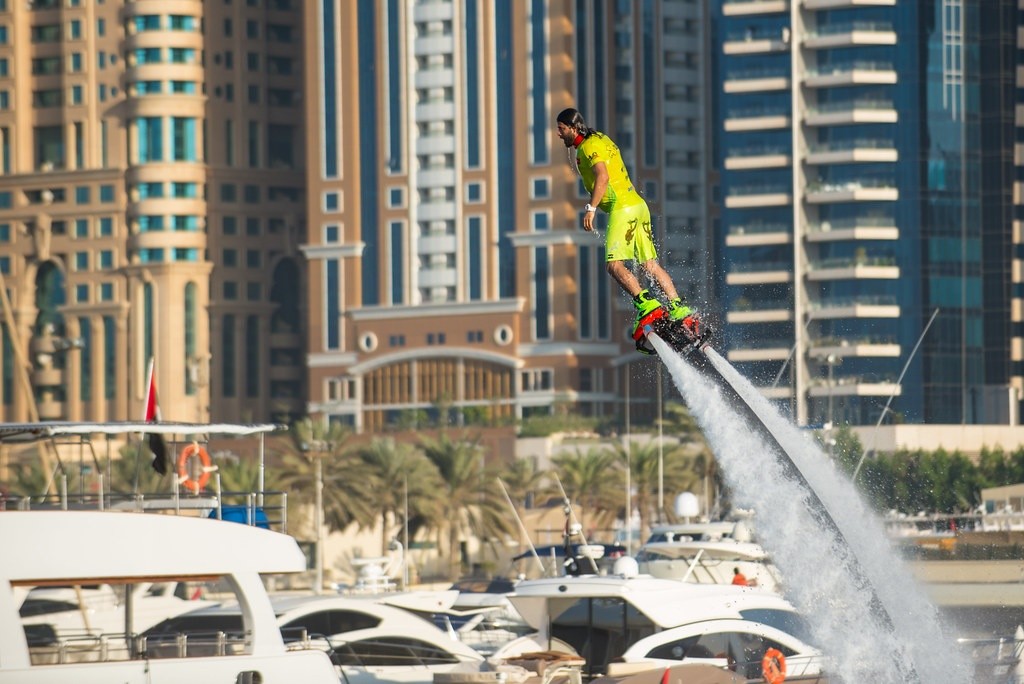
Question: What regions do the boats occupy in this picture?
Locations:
[0,308,1024,684]
[0,510,345,684]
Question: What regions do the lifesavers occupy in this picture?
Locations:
[178,445,212,493]
[762,648,787,682]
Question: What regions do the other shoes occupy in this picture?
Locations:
[662,297,712,358]
[632,289,676,355]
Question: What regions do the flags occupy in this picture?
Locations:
[140,372,166,476]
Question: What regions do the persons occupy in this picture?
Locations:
[557,107,700,341]
[731,567,746,586]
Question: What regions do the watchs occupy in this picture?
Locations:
[584,204,597,212]
